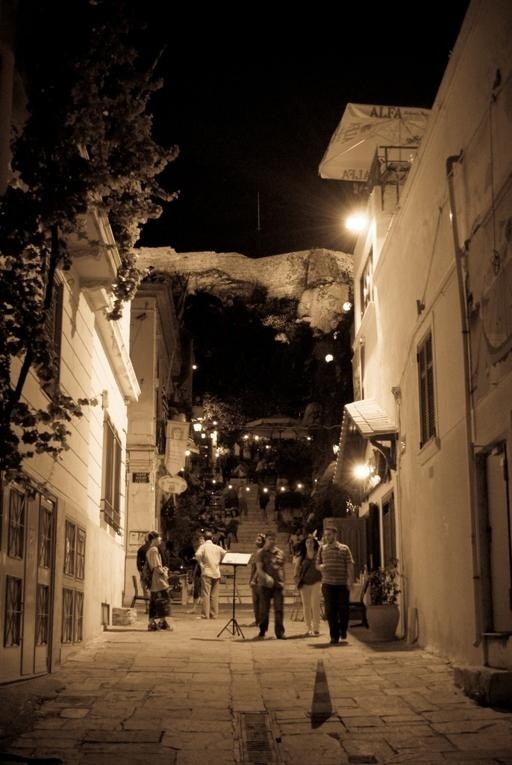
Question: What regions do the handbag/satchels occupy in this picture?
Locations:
[295,575,303,589]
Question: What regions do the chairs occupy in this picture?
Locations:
[130,576,150,613]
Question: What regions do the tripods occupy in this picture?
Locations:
[217,567,245,639]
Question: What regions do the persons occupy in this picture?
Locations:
[255,458,272,483]
[184,484,355,646]
[136,529,171,631]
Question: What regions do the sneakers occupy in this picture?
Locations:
[259,631,287,639]
[148,621,173,631]
[306,631,349,646]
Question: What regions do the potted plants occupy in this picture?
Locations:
[365,559,404,642]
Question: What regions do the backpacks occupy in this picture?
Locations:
[140,561,155,591]
